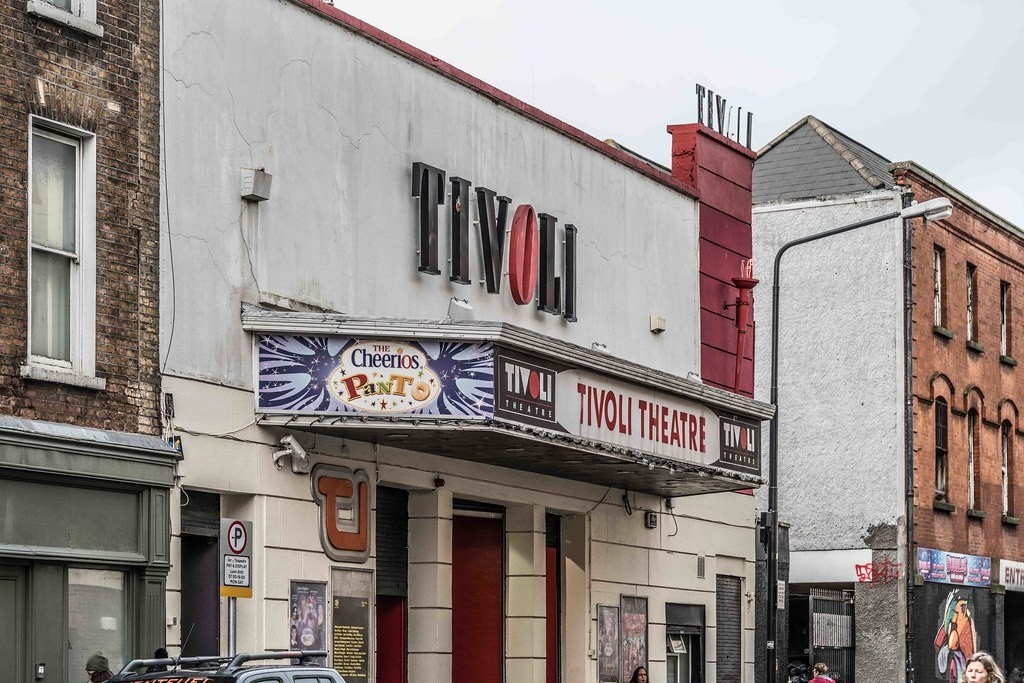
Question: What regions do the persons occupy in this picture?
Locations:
[145,648,169,673]
[807,663,836,683]
[291,594,324,649]
[85,650,112,683]
[961,652,1004,683]
[629,666,650,683]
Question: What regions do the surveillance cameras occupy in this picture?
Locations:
[280,435,306,460]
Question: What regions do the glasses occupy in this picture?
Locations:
[87,673,93,676]
[812,670,816,673]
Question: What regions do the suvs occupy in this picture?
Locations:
[106,648,345,683]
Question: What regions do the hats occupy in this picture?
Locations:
[86,650,110,671]
[154,648,168,659]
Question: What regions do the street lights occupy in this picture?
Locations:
[766,196,951,683]
